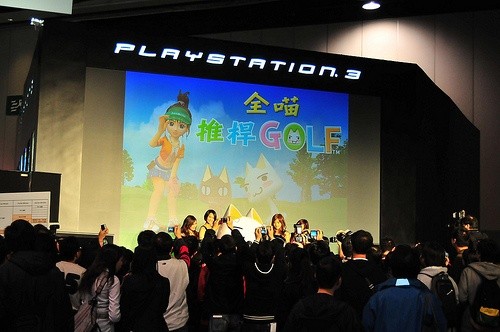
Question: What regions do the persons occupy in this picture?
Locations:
[290,219,309,244]
[462,215,488,241]
[272,214,291,242]
[181,215,199,237]
[198,210,217,239]
[0,219,500,332]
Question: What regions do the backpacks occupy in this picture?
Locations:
[420,271,457,310]
[467,266,500,329]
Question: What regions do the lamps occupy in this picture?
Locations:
[362,0,380,10]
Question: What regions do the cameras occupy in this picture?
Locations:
[311,231,317,236]
[296,225,302,235]
[101,224,106,231]
[330,233,346,243]
[453,210,465,223]
[168,227,174,232]
[260,227,268,234]
[221,218,227,223]
